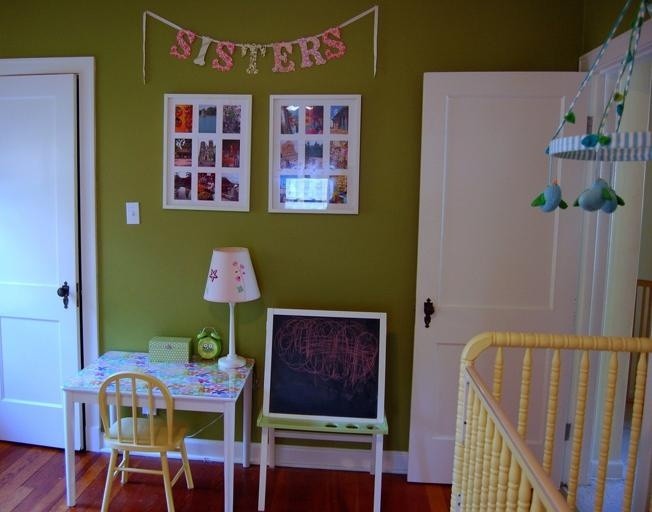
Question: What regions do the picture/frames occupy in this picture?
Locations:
[162,94,252,213]
[268,94,361,215]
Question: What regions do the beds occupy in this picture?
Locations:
[451,330,652,512]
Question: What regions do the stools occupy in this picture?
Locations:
[257,408,389,512]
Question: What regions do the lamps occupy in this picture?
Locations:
[203,247,261,369]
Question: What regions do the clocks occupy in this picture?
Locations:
[193,327,222,361]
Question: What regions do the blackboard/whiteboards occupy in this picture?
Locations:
[263,308,387,424]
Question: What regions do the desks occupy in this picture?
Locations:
[61,351,255,512]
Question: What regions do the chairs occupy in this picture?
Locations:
[99,372,195,512]
